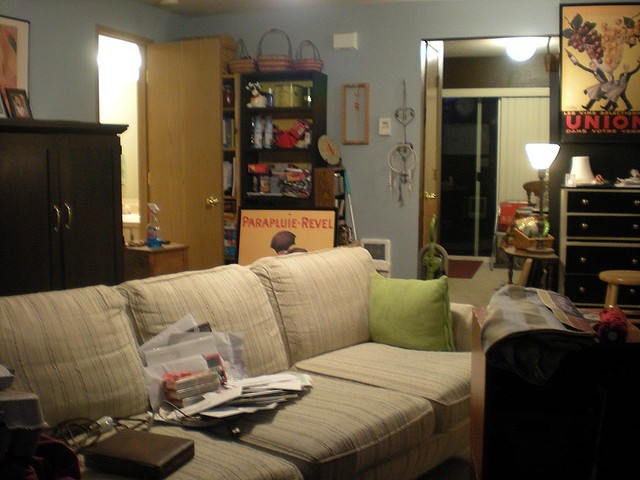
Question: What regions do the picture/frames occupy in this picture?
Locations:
[4,88,34,118]
[558,2,640,146]
[0,14,30,116]
[1,90,10,118]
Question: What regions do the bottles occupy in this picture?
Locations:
[252,118,262,148]
[264,115,274,148]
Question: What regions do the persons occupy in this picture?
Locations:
[576,61,619,112]
[600,62,640,111]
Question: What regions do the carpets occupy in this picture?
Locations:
[444,257,483,280]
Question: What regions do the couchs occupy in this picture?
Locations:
[1,246,476,480]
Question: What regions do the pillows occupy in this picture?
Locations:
[369,272,459,352]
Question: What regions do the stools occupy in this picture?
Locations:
[504,246,559,289]
[599,268,639,321]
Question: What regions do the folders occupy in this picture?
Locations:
[141,313,314,430]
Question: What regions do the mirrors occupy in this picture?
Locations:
[341,82,371,145]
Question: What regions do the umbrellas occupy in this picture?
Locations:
[270,231,296,253]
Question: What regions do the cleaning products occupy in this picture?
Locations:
[145,202,160,249]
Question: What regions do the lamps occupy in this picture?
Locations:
[526,143,561,253]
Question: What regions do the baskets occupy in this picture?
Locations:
[258,29,293,72]
[228,38,257,74]
[294,40,323,72]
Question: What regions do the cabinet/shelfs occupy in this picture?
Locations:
[242,70,328,211]
[557,189,640,308]
[0,118,128,297]
[124,240,190,282]
[222,74,240,271]
